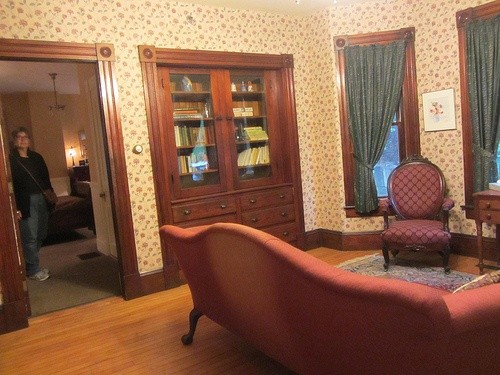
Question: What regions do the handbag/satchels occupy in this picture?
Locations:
[44,190,57,213]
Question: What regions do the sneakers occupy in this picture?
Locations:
[29,268,50,281]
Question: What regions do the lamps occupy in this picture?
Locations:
[47,72,65,111]
[68,146,76,167]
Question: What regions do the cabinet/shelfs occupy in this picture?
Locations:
[73,165,91,182]
[138,45,309,290]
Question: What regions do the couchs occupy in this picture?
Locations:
[158,222,500,374]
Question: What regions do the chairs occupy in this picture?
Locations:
[379,153,455,275]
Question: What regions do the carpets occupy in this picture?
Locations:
[334,252,487,294]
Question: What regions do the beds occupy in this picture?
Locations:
[47,183,97,236]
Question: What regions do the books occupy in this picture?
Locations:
[178,156,193,174]
[173,108,203,118]
[174,125,214,146]
[237,145,270,166]
[233,107,253,117]
[243,126,269,140]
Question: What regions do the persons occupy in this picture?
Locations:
[9,127,53,281]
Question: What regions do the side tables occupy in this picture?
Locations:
[472,189,500,275]
